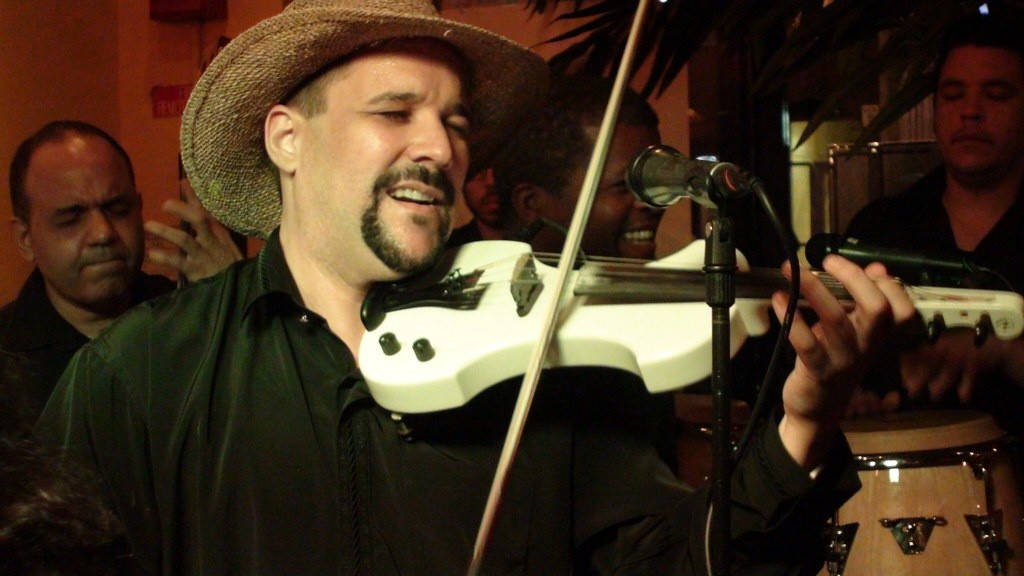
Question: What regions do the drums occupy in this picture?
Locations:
[813,406,1023,576]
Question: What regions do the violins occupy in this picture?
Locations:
[354,236,1024,423]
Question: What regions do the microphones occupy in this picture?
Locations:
[625,144,747,210]
[805,231,993,280]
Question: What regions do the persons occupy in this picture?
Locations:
[32,0,928,575]
[0,120,252,576]
[839,12,1024,416]
[443,75,677,463]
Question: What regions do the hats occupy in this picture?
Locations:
[181,2,552,244]
